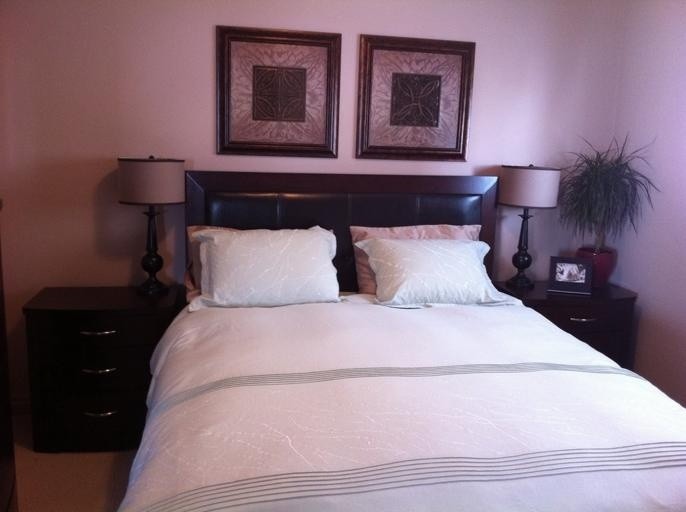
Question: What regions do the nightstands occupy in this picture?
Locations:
[496,279,638,369]
[22,286,182,454]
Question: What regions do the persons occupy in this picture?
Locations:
[556,263,585,283]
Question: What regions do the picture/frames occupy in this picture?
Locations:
[214,22,342,158]
[546,256,594,295]
[355,31,475,161]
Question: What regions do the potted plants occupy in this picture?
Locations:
[557,133,661,288]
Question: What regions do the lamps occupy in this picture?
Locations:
[114,155,185,298]
[492,164,562,294]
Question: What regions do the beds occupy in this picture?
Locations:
[118,168,684,511]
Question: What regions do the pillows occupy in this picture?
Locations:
[349,223,483,295]
[183,222,240,303]
[353,238,521,308]
[189,225,340,312]
